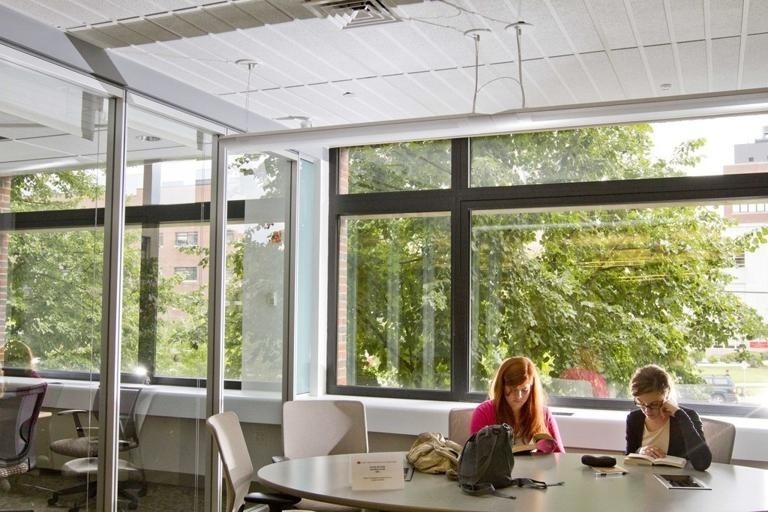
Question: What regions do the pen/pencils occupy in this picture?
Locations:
[595,471,630,478]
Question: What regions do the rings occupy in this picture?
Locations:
[650,447,654,451]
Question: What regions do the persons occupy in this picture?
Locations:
[619,362,711,472]
[470,356,565,453]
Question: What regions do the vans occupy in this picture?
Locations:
[673,376,737,404]
[712,345,742,356]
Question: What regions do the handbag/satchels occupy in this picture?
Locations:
[405,432,463,482]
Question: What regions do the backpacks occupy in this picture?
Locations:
[457,425,565,501]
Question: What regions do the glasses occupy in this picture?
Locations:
[633,397,667,410]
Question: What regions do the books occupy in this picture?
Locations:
[592,466,629,474]
[653,473,713,490]
[623,450,687,468]
[509,430,553,454]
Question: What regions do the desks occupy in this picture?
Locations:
[255,451,767,512]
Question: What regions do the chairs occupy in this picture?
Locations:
[0,382,155,512]
[698,415,734,464]
[448,408,476,449]
[205,399,370,512]
